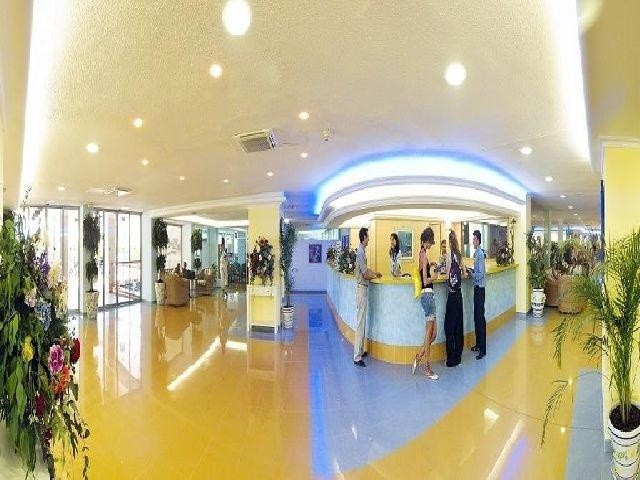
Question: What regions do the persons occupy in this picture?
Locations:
[443,231,467,367]
[411,227,440,382]
[387,233,405,278]
[220,237,230,298]
[466,229,487,361]
[438,240,446,273]
[352,227,384,367]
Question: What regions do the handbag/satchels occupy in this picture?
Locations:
[410,262,422,299]
[448,262,461,291]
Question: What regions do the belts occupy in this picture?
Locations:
[421,288,434,293]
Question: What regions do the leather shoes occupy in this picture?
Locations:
[362,351,367,357]
[476,351,485,360]
[470,344,480,351]
[354,360,366,367]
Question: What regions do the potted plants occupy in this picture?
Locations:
[277,224,297,329]
[524,227,640,480]
[81,211,101,320]
[152,218,168,305]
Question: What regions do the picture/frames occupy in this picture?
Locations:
[309,243,323,264]
[396,230,413,260]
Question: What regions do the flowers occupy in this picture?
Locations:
[246,235,276,286]
[1,182,92,480]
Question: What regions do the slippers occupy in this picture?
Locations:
[426,372,439,380]
[411,352,418,375]
